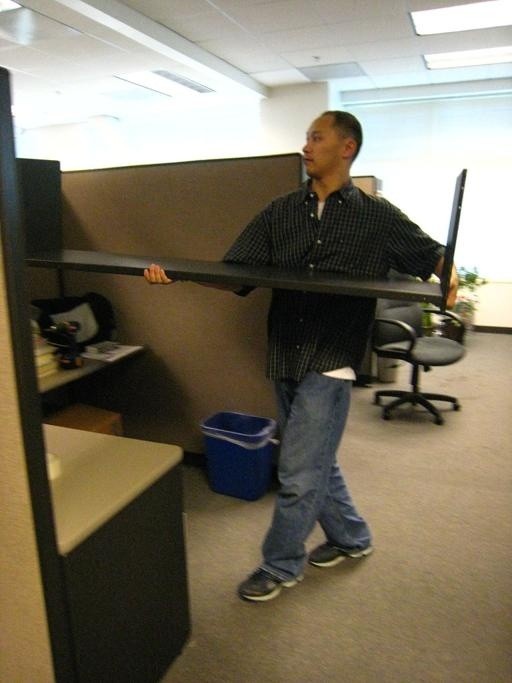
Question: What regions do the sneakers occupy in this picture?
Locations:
[239,571,304,602]
[308,544,374,567]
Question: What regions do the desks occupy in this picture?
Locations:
[35,339,192,682]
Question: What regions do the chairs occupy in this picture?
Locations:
[372,268,468,425]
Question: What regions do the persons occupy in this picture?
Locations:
[142,110,460,602]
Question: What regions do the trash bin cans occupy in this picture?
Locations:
[200,412,277,502]
[377,356,406,383]
